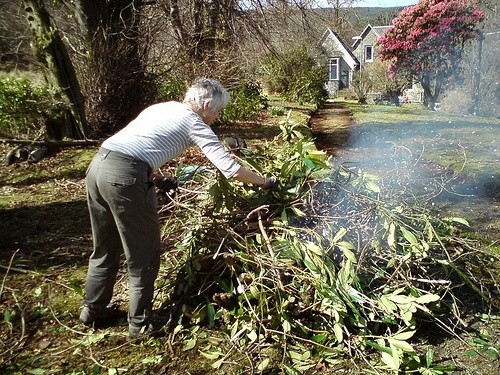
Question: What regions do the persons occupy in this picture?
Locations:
[79,77,279,337]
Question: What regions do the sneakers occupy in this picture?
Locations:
[128,310,169,338]
[79,305,119,328]
